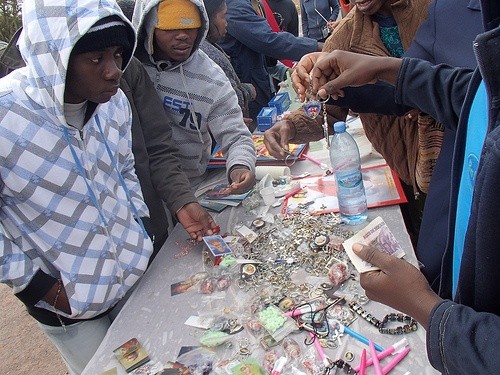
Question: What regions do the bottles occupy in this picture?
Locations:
[330,122,369,226]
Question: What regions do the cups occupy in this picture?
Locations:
[260,187,275,205]
[321,26,328,38]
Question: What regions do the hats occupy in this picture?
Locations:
[72,16,130,55]
[145,0,202,56]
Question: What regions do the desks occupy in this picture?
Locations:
[82,114,443,375]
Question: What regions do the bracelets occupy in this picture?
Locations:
[326,360,357,375]
[53,278,62,308]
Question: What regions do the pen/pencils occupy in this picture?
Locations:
[278,185,307,200]
[302,153,329,169]
[352,338,412,375]
[339,324,385,352]
[309,330,330,368]
[284,304,317,318]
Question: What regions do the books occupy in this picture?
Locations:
[281,164,408,216]
[207,139,309,170]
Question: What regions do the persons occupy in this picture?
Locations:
[300,0,340,43]
[264,0,298,37]
[290,0,483,285]
[311,1,500,375]
[263,1,444,248]
[0,26,220,257]
[327,0,353,33]
[219,0,326,134]
[123,0,255,235]
[198,0,257,129]
[0,0,156,375]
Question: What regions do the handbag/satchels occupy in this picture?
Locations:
[415,111,445,194]
